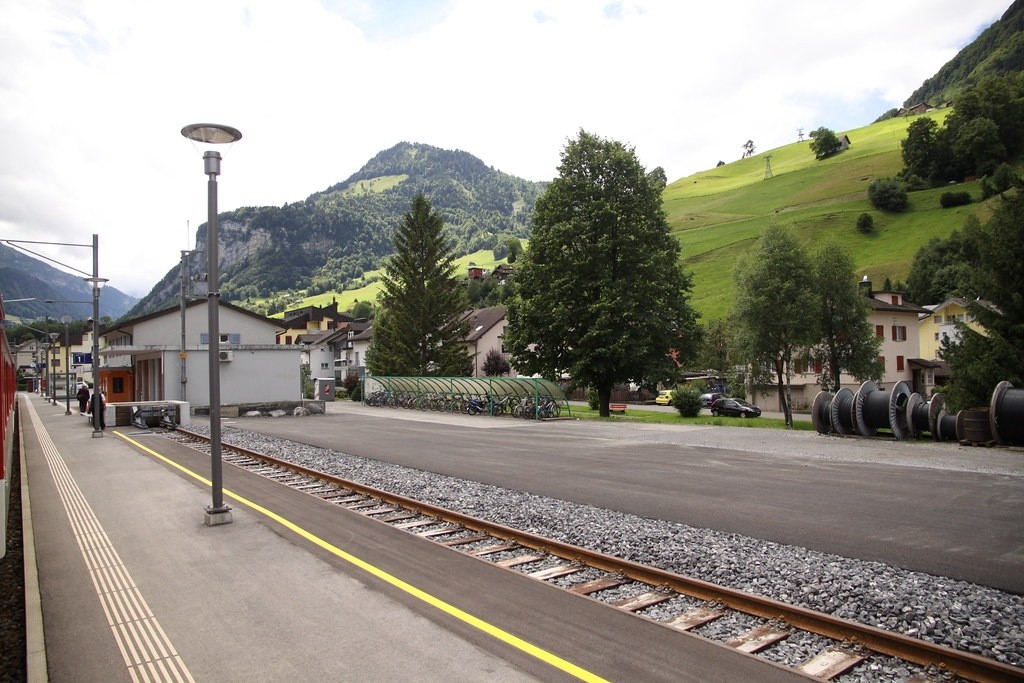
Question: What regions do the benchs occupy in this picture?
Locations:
[609,403,627,413]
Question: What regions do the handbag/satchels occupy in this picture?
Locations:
[78,393,83,400]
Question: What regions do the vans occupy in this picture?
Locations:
[701,393,720,408]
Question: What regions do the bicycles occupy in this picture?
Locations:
[364,388,562,419]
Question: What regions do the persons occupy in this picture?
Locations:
[76,384,90,416]
[86,387,106,431]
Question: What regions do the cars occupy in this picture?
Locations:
[710,398,762,418]
[655,391,675,406]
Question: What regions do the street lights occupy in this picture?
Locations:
[178,122,244,528]
[33,342,51,401]
[61,315,72,416]
[49,332,61,407]
[82,276,110,438]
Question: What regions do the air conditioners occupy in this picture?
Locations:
[219,351,233,361]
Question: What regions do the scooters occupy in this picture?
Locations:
[466,391,496,415]
